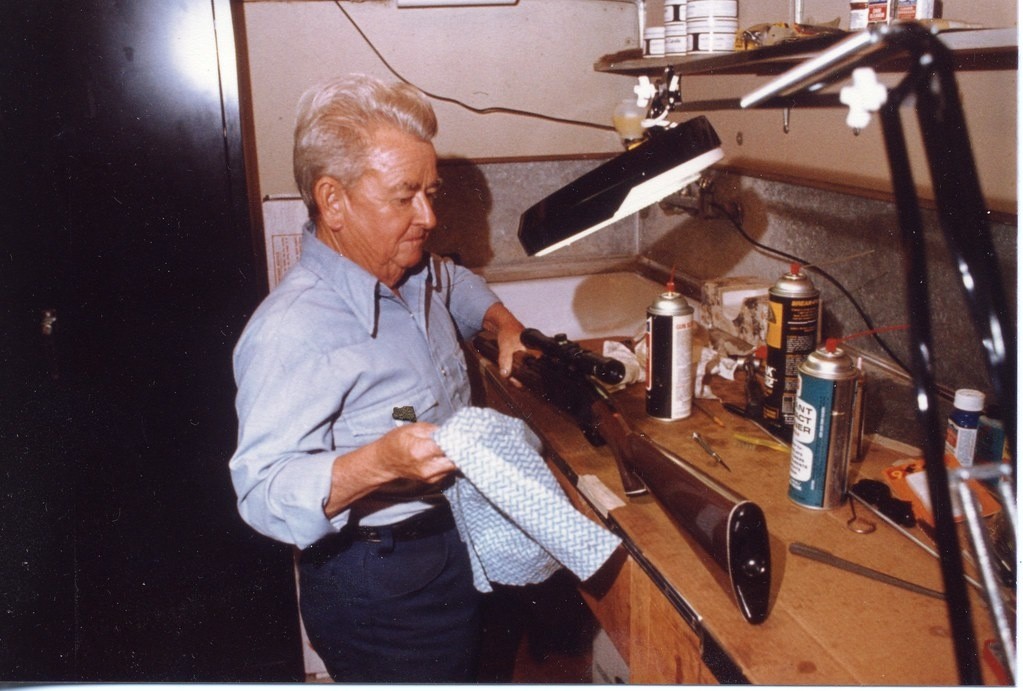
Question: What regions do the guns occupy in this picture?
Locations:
[472,327,770,626]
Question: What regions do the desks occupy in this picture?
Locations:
[461,334,1016,685]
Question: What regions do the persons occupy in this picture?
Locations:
[228,79,528,684]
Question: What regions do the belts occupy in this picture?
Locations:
[335,512,454,541]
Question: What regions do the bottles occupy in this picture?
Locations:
[946,388,986,469]
[788,337,863,509]
[645,283,695,422]
[762,264,823,432]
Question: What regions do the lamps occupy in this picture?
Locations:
[516,118,729,259]
